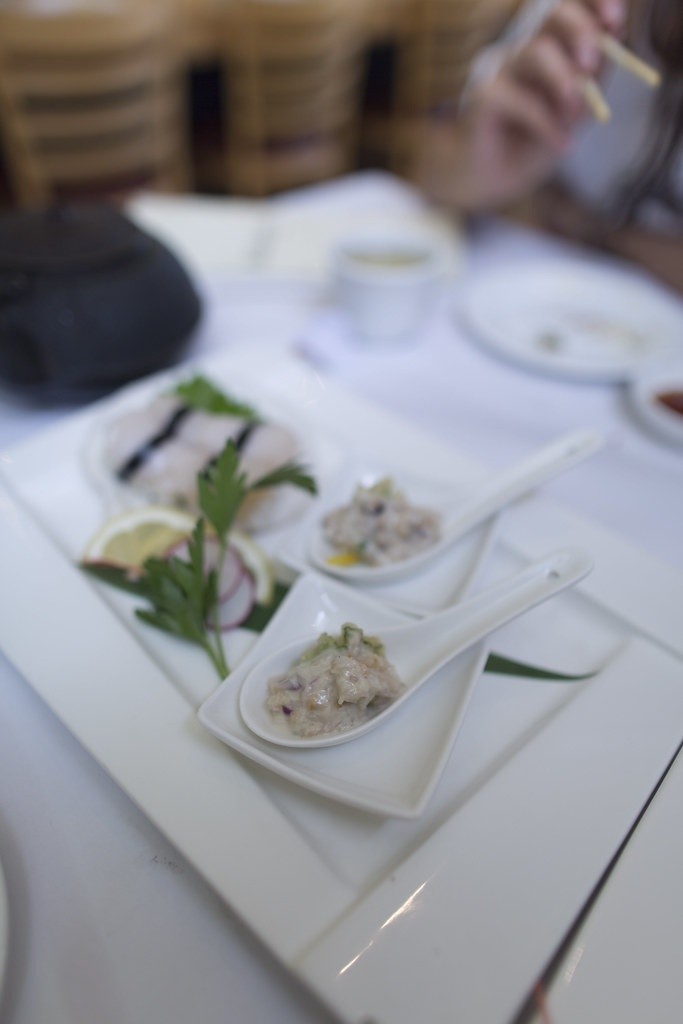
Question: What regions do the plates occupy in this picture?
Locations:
[193,468,643,829]
[454,247,682,443]
[1,340,682,1024]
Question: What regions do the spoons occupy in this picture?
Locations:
[304,425,602,580]
[240,548,597,747]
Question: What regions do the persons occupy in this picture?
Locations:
[386,0,683,293]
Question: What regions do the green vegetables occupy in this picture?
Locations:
[81,372,317,683]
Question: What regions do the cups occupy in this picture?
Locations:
[333,222,438,361]
